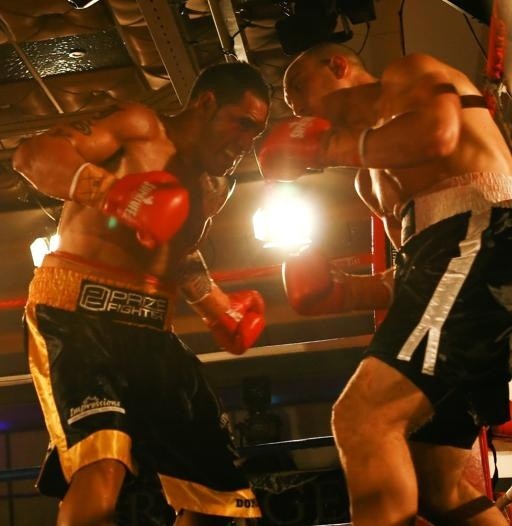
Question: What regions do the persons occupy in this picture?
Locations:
[253,42,512,525]
[11,60,269,526]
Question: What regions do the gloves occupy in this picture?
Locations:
[101,171,190,250]
[202,291,265,354]
[281,245,352,316]
[253,114,331,184]
